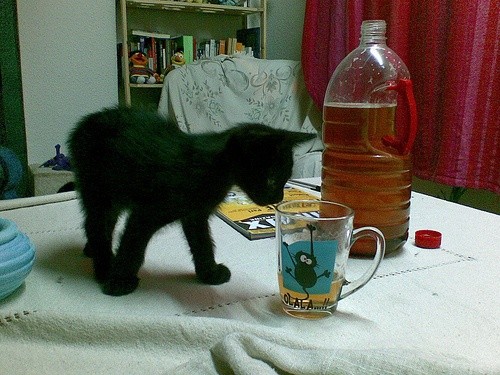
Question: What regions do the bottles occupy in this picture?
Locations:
[320,20,417,258]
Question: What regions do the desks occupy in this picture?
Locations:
[0,177,500,375]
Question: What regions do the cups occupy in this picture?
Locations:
[275,199,385,320]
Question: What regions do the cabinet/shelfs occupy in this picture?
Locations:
[120,0,267,108]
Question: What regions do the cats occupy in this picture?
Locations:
[66,100,319,297]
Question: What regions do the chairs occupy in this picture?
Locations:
[158,53,323,178]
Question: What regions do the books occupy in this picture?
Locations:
[126,28,253,83]
[212,180,325,240]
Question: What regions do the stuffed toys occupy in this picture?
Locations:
[128,51,161,84]
[160,51,185,82]
